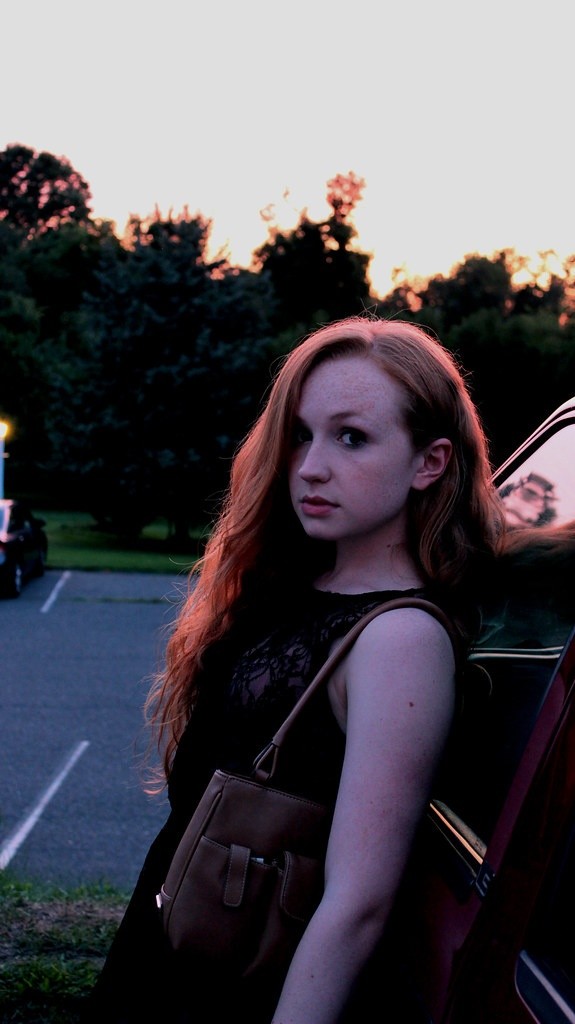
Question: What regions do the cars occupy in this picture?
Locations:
[395,392,575,1023]
[0,499,49,599]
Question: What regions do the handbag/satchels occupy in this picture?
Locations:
[154,597,455,981]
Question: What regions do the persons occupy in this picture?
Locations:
[85,316,504,1023]
